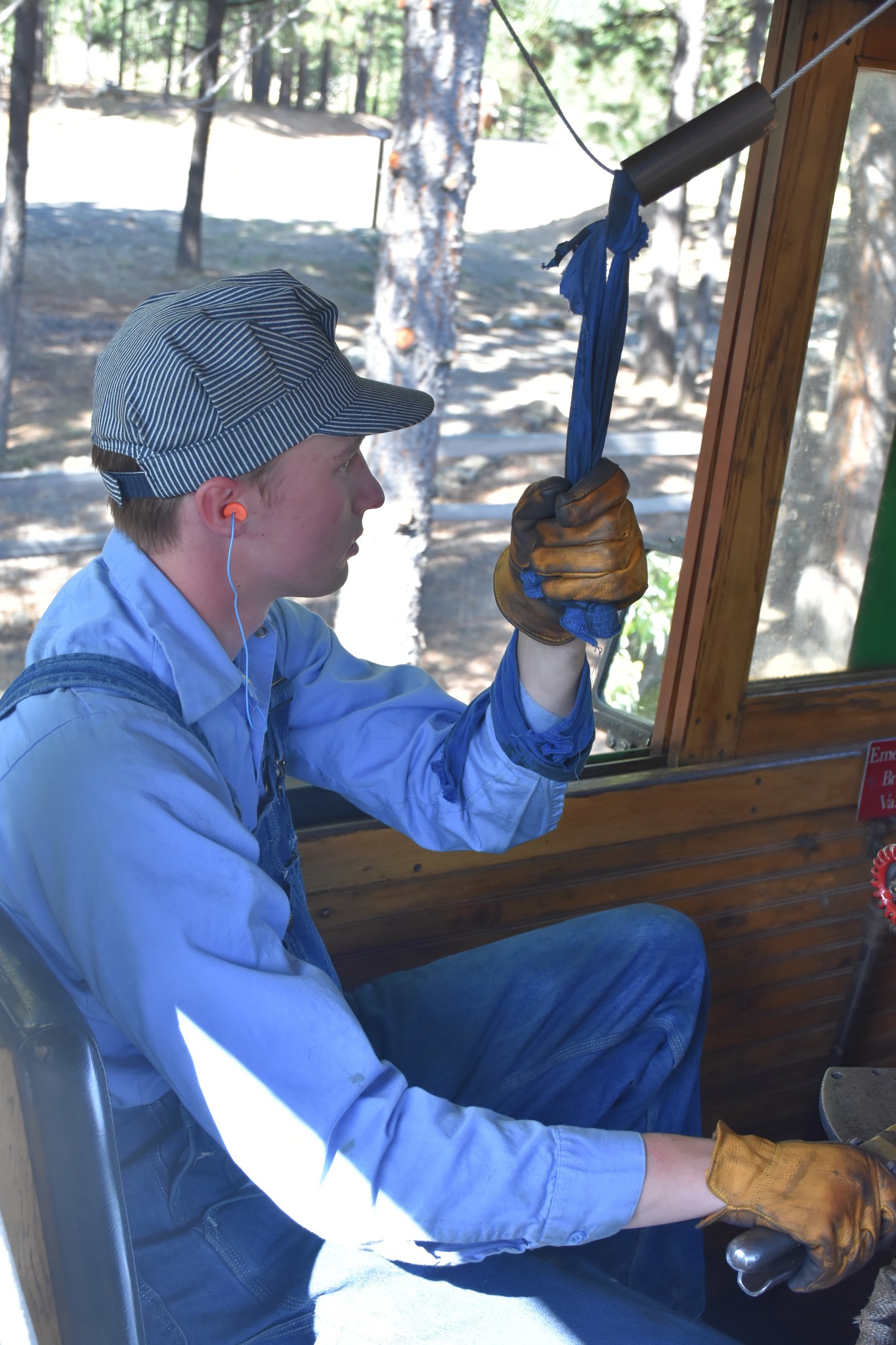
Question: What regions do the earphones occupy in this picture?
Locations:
[223,502,247,522]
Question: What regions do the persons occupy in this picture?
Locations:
[0,269,896,1345]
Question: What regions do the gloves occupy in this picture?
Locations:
[694,1121,896,1293]
[493,456,647,646]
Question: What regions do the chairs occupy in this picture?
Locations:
[0,910,147,1345]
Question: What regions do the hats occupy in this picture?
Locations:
[89,266,437,505]
